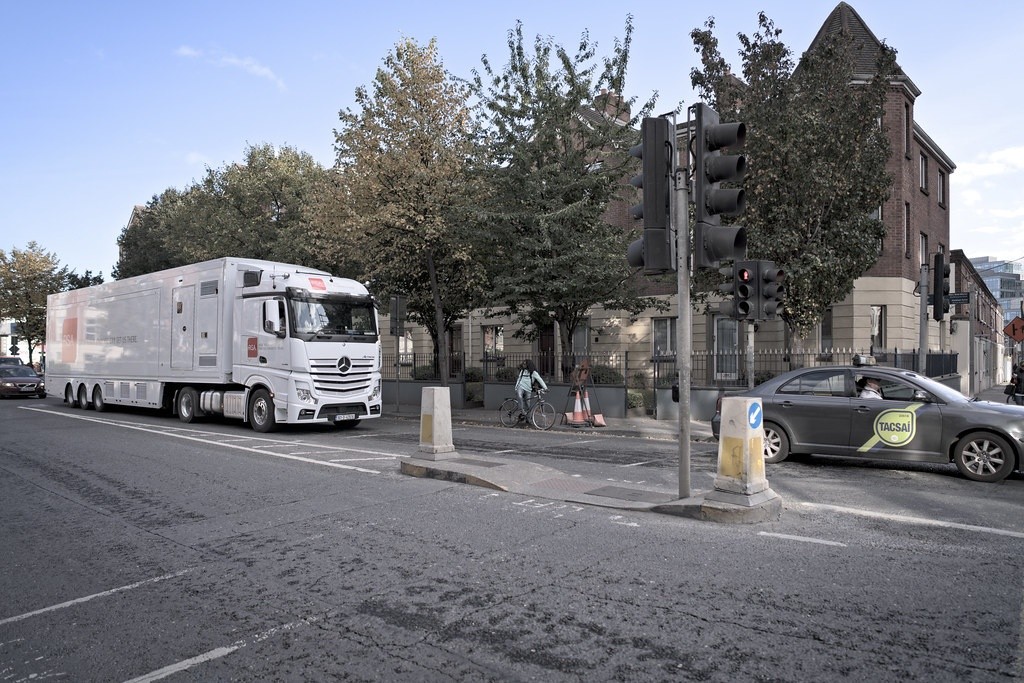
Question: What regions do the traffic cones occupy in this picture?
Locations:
[572,381,591,428]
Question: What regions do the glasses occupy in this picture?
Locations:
[870,378,882,381]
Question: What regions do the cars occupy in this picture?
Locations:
[710,354,1023,484]
[0,364,47,401]
[0,355,25,366]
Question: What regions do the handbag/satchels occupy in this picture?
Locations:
[1005,380,1016,395]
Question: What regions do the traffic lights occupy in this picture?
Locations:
[627,112,678,276]
[732,259,759,321]
[760,261,787,324]
[933,252,951,322]
[686,100,751,270]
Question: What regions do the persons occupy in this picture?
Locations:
[1011,361,1024,405]
[1012,363,1018,370]
[514,359,550,426]
[858,376,884,400]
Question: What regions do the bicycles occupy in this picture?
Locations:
[499,384,556,432]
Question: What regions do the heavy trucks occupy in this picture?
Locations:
[40,255,382,434]
